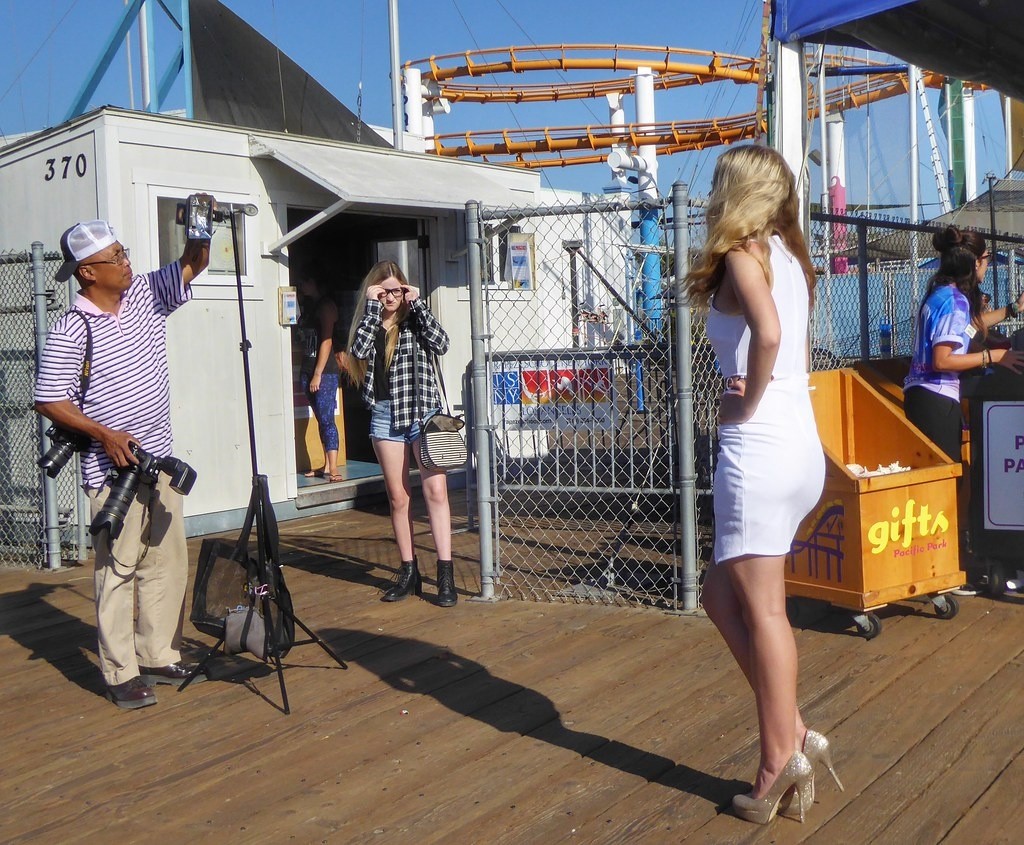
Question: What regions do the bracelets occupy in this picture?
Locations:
[1012,302,1018,318]
[981,349,987,369]
[986,348,992,363]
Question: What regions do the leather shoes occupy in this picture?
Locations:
[105,677,158,709]
[138,662,207,685]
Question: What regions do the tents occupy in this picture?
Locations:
[769,0,1023,106]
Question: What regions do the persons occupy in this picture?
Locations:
[672,144,845,826]
[335,254,373,318]
[901,225,1024,546]
[31,192,218,710]
[346,260,459,608]
[295,262,347,484]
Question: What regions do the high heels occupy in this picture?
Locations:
[776,729,845,815]
[732,753,812,824]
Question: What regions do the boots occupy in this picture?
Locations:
[384,555,422,600]
[434,558,459,606]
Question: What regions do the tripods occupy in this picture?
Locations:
[176,208,350,715]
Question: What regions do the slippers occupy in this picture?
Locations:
[329,473,343,483]
[305,469,332,478]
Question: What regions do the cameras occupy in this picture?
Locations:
[88,441,197,539]
[36,423,91,479]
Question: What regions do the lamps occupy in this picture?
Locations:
[176,195,258,239]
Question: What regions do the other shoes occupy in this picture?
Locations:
[951,583,985,595]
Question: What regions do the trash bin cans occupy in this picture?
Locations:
[784,368,965,639]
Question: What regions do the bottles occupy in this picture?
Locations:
[879,324,892,359]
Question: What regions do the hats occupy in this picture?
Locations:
[53,220,117,282]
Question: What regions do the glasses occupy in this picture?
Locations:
[76,248,130,268]
[378,287,406,297]
[978,252,993,262]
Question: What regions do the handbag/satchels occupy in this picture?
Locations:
[420,414,469,472]
[190,475,294,658]
[224,608,271,662]
